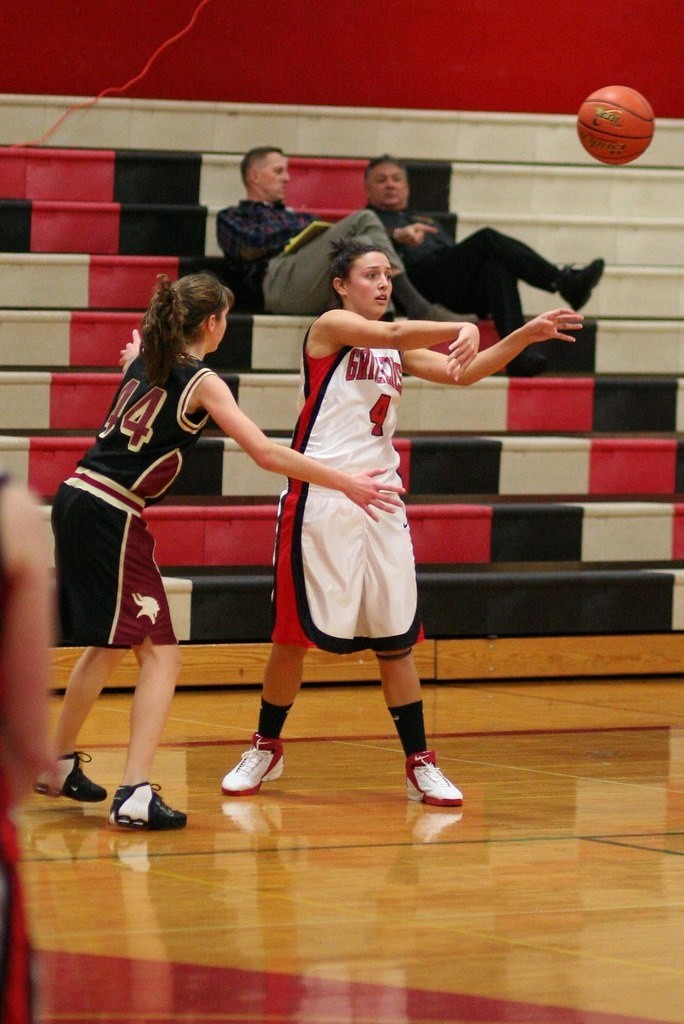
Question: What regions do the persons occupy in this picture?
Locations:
[34,272,406,831]
[0,470,61,1024]
[216,146,604,378]
[221,237,586,808]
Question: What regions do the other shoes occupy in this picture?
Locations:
[558,257,605,311]
[506,349,547,377]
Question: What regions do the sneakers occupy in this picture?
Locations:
[33,751,108,802]
[405,750,464,807]
[109,782,188,829]
[221,732,284,796]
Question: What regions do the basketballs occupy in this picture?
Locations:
[576,84,660,169]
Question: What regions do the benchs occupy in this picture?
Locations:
[0,94,684,688]
